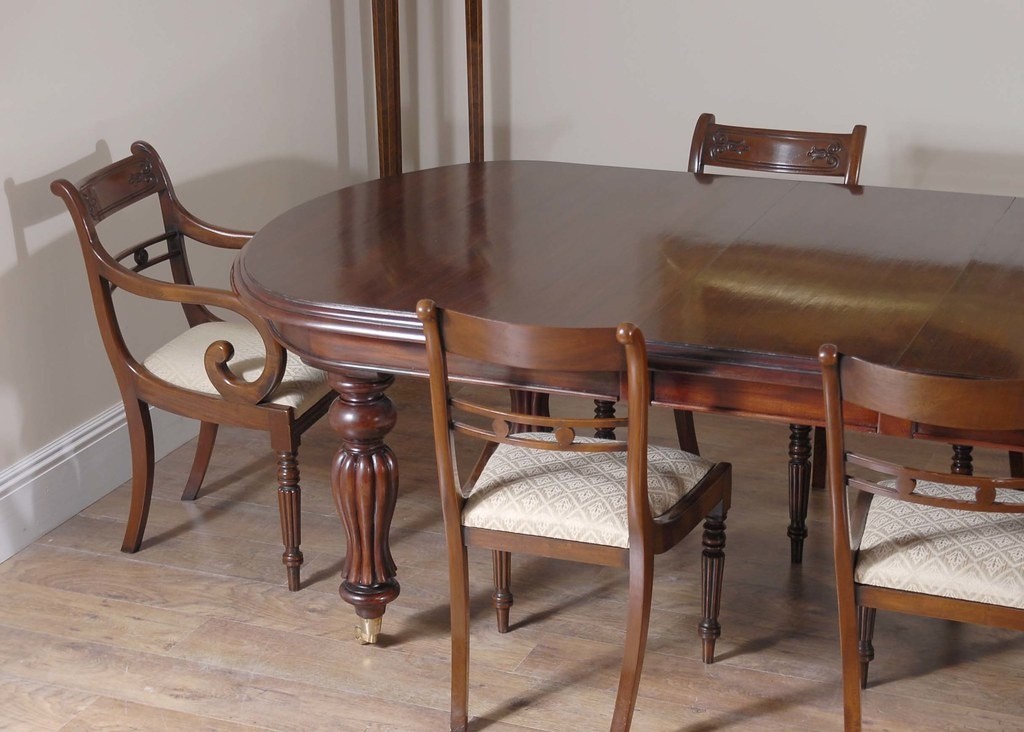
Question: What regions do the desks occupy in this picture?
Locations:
[229,159,1023,647]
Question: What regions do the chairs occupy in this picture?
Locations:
[596,112,868,490]
[49,139,339,592]
[415,297,733,732]
[818,343,1024,732]
